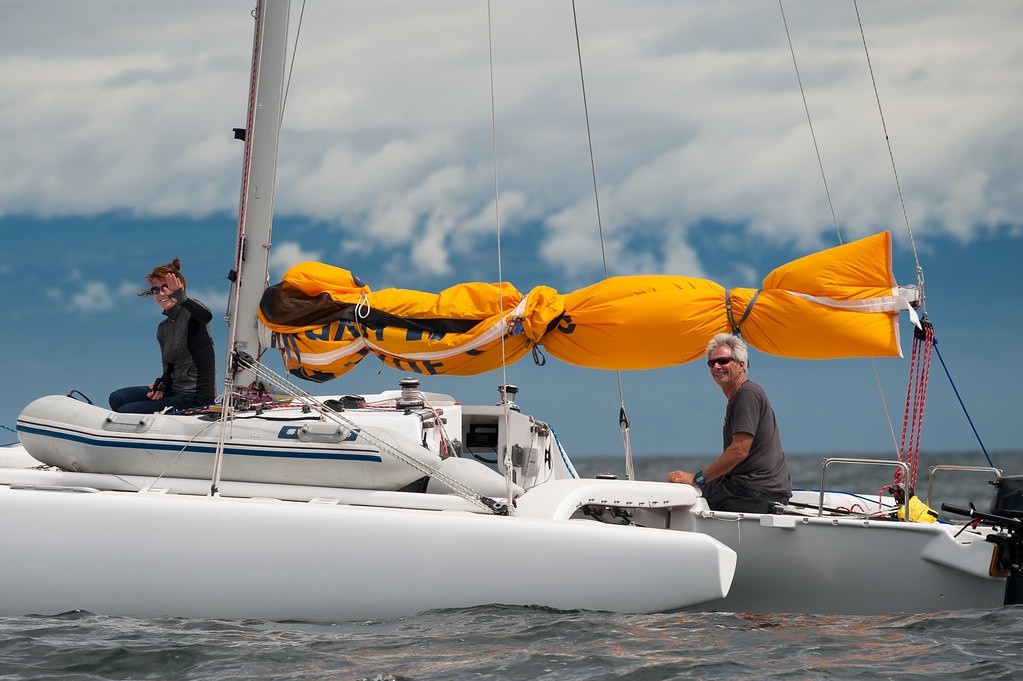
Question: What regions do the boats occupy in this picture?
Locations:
[13,389,527,500]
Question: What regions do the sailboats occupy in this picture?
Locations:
[0,0,1023,626]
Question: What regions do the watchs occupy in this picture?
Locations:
[694,472,706,485]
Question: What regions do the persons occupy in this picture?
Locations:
[109,258,215,415]
[667,332,792,514]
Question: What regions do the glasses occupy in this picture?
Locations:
[707,357,737,368]
[150,283,169,296]
